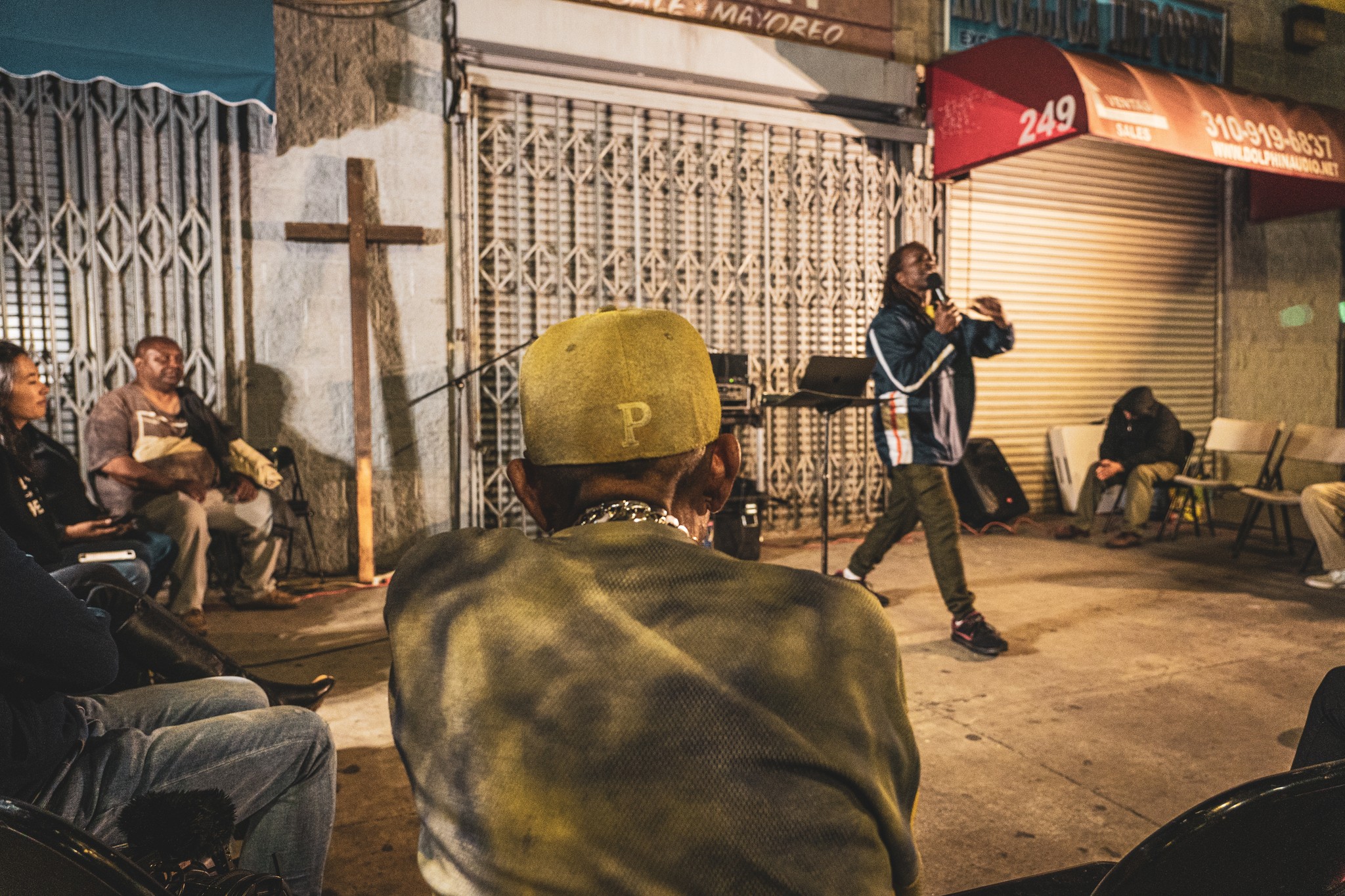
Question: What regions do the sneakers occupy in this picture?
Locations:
[834,569,890,607]
[950,610,1009,655]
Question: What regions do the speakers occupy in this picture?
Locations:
[945,437,1030,530]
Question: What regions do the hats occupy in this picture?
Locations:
[520,303,722,468]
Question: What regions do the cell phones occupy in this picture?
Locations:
[77,550,137,564]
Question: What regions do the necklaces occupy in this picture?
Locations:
[570,499,700,543]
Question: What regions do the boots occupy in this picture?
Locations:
[116,594,336,710]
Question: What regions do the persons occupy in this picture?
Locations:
[0,526,338,896]
[383,307,927,896]
[1054,383,1185,550]
[832,240,1015,655]
[0,337,336,713]
[1299,481,1345,590]
[84,335,306,636]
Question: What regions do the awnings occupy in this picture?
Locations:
[931,31,1345,224]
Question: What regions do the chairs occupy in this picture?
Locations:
[937,757,1345,896]
[0,798,288,896]
[89,445,323,602]
[1104,416,1345,570]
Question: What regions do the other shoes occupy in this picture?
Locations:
[1055,524,1092,539]
[181,609,210,637]
[1106,531,1142,547]
[1304,569,1345,590]
[238,591,301,606]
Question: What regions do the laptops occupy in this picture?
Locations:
[798,356,873,396]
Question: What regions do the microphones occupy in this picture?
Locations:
[926,272,947,305]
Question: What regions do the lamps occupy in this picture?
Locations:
[453,42,482,88]
